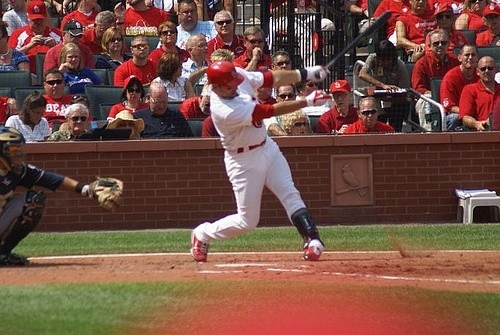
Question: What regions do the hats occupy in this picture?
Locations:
[207,60,245,90]
[26,0,86,36]
[105,75,145,133]
[482,3,500,17]
[329,80,352,94]
[434,2,453,16]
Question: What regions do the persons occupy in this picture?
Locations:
[0,0,500,139]
[190,60,332,262]
[0,127,124,266]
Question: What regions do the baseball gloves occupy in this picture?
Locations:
[86,177,124,211]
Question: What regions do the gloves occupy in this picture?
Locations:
[305,89,332,106]
[295,65,331,83]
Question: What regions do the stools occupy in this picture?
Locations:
[457,196,500,225]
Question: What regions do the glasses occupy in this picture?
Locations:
[359,108,378,116]
[479,67,496,72]
[278,94,294,99]
[116,120,136,127]
[293,122,310,128]
[436,14,452,20]
[431,40,450,47]
[127,87,170,105]
[44,34,88,121]
[110,29,177,50]
[246,39,291,66]
[216,19,232,25]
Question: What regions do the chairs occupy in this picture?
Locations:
[0,0,500,143]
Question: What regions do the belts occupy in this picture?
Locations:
[237,136,267,153]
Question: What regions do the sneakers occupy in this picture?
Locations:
[302,238,325,262]
[0,251,30,269]
[190,230,208,262]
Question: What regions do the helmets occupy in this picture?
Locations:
[0,126,28,177]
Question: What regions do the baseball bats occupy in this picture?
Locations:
[315,10,392,79]
